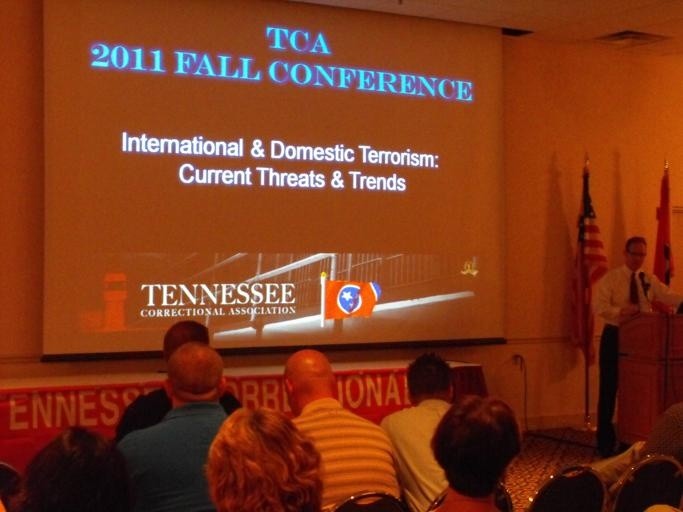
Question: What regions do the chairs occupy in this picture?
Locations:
[0,459,21,498]
[421,483,514,512]
[517,460,606,512]
[606,450,682,511]
[324,487,408,512]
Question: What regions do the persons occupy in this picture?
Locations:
[591,237,683,458]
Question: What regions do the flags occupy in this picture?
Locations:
[568,168,610,368]
[325,279,382,320]
[651,171,675,313]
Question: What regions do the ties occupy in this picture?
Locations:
[629,271,639,305]
